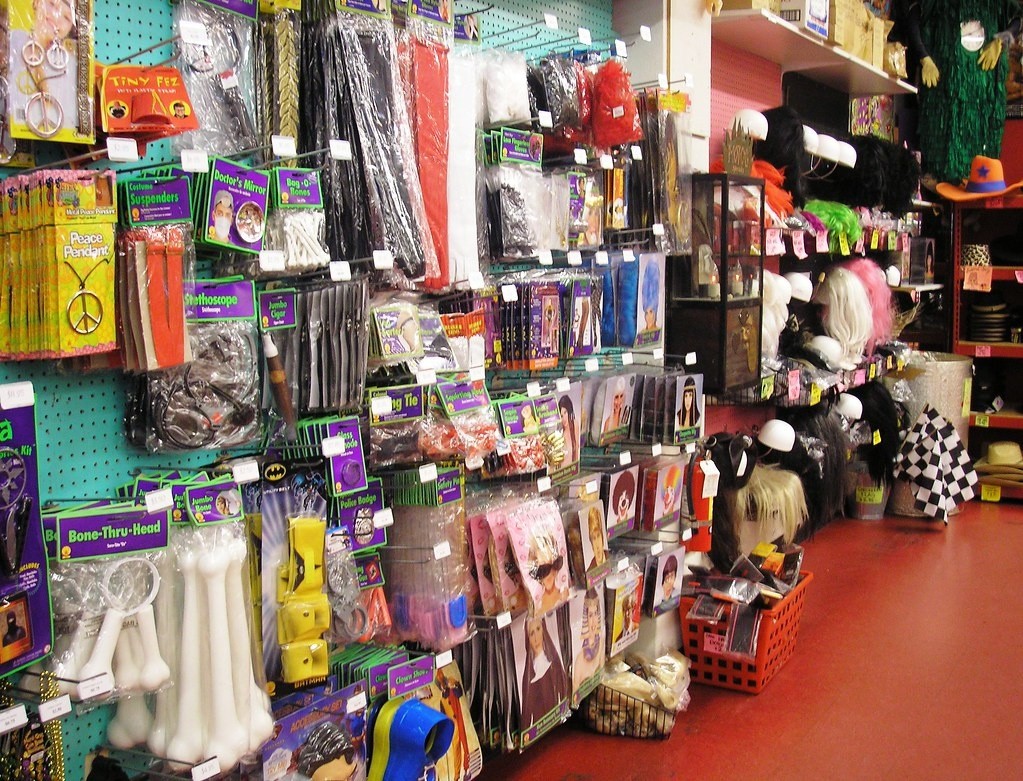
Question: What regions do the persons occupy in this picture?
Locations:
[174,103,187,118]
[604,377,630,432]
[210,190,235,245]
[532,137,541,160]
[522,614,567,728]
[642,306,659,330]
[574,588,604,689]
[10,0,79,127]
[591,277,603,346]
[616,595,639,642]
[549,396,579,472]
[110,101,126,118]
[577,296,590,348]
[435,669,472,781]
[657,555,680,606]
[579,177,585,197]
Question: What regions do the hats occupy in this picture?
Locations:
[972,441,1023,488]
[935,154,1023,202]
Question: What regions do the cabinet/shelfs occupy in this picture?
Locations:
[952,193,1023,501]
[709,8,945,409]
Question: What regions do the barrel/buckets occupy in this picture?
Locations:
[903,351,973,433]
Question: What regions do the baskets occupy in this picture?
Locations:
[586,682,678,742]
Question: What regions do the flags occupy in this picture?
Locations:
[893,407,979,526]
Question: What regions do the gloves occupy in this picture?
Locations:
[977,38,1002,72]
[919,56,940,88]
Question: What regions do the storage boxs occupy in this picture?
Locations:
[780,0,908,78]
[679,570,814,696]
